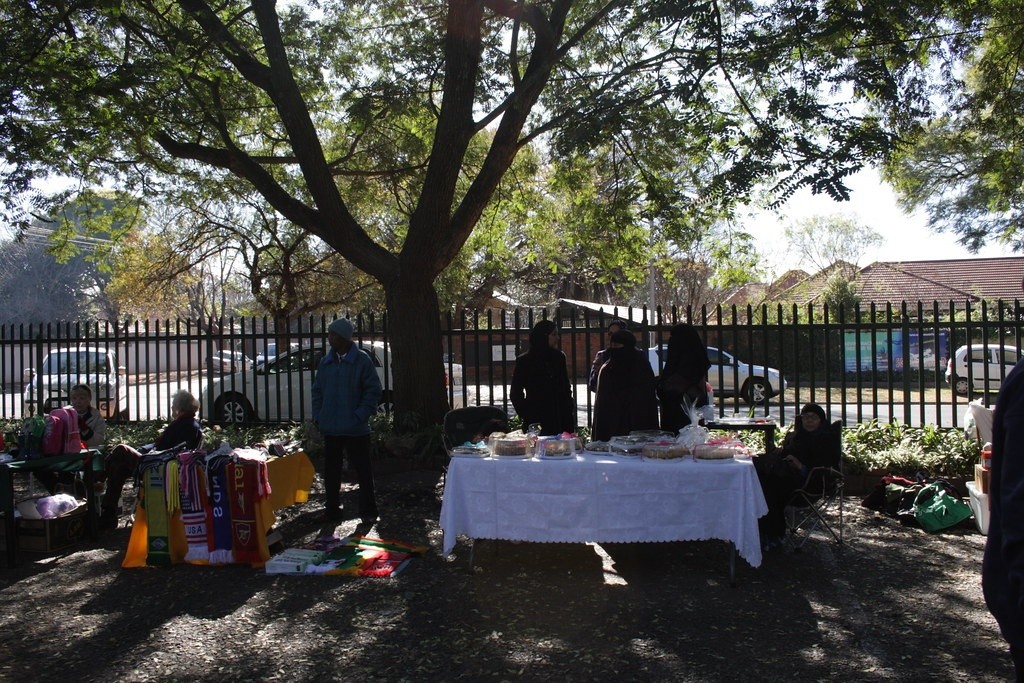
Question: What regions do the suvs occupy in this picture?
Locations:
[642,342,788,408]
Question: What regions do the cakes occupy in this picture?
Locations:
[494,434,733,461]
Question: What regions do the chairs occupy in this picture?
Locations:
[442,406,511,493]
[781,420,847,556]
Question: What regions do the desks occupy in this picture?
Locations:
[261,447,314,525]
[0,446,100,565]
[439,447,771,581]
[705,416,777,450]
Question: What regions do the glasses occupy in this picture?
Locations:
[801,414,820,421]
[71,384,90,392]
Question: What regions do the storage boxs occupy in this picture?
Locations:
[14,507,89,553]
[965,451,993,534]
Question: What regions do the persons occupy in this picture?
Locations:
[35,385,106,496]
[657,323,711,434]
[589,320,626,393]
[93,390,202,532]
[759,404,834,547]
[982,357,1024,682]
[510,319,575,435]
[312,318,380,523]
[591,331,657,442]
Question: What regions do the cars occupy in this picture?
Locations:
[944,342,1024,397]
[213,348,253,375]
[197,338,469,428]
[24,346,130,426]
[255,342,304,366]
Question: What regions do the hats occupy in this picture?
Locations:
[327,317,354,342]
[801,403,826,424]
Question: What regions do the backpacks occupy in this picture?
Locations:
[14,405,83,460]
